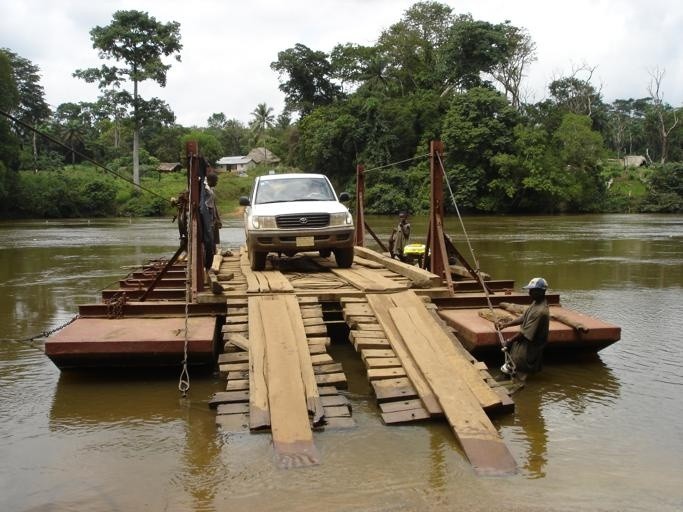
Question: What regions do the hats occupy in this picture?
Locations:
[522,277,548,289]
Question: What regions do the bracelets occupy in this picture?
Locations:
[506,339,511,345]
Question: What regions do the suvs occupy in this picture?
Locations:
[235,172,357,273]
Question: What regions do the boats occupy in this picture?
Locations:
[399,239,431,260]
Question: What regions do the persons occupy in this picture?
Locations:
[198,156,222,291]
[389,210,410,263]
[495,277,549,376]
